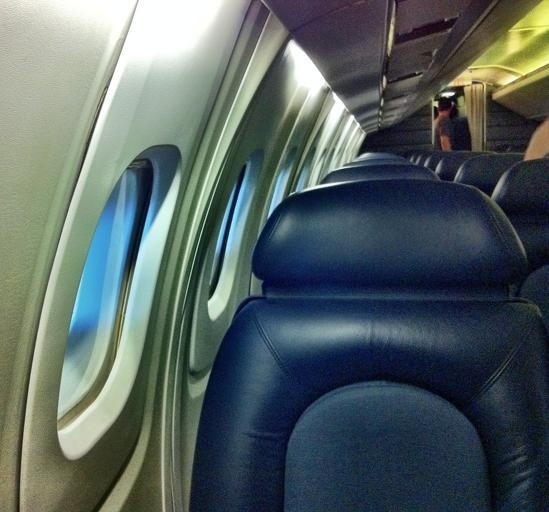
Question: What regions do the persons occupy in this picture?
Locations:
[435,95,461,151]
